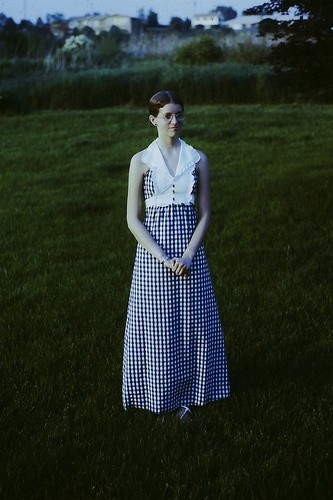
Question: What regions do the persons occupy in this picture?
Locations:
[119,89,231,422]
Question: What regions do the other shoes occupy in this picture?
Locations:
[177,404,194,426]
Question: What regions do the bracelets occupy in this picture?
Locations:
[158,256,171,267]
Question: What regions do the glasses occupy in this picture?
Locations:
[155,112,186,121]
